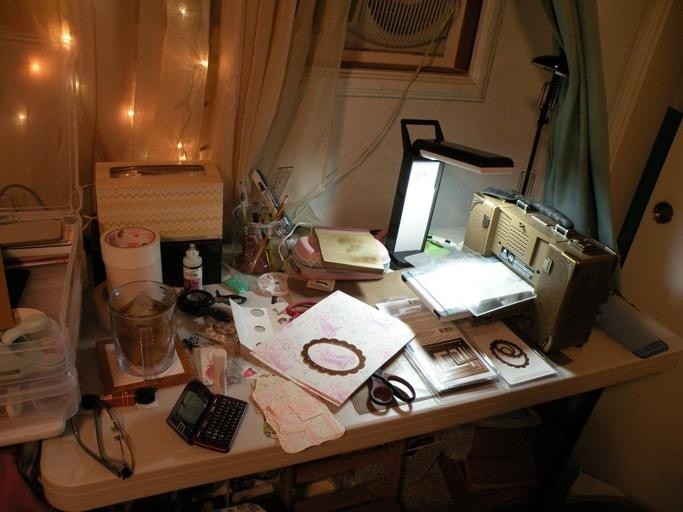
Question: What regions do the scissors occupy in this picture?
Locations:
[286,299,320,317]
[369,368,417,406]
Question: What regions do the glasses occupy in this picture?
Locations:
[70,402,135,479]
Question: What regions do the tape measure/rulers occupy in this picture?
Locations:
[259,166,294,224]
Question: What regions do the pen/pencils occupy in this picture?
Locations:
[252,213,271,267]
[240,180,248,256]
[249,194,288,274]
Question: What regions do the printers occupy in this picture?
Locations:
[401,186,621,353]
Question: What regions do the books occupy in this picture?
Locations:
[312,225,385,274]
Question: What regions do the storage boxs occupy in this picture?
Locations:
[282,425,571,511]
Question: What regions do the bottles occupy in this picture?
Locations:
[182,242,203,291]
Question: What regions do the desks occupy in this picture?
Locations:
[39,238,682,511]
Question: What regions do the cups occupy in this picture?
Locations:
[233,204,286,275]
[107,281,177,377]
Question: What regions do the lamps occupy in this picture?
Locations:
[521,54,568,196]
[387,119,513,265]
